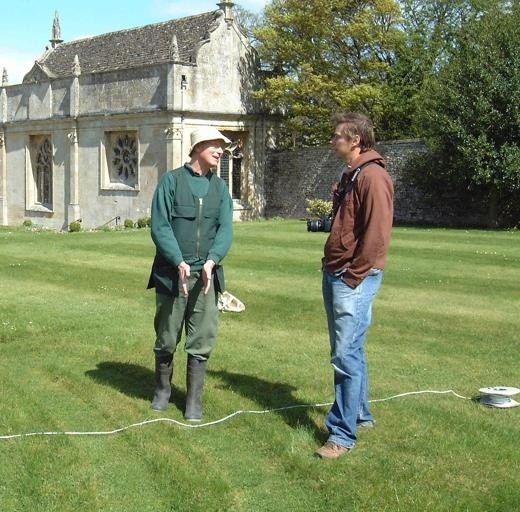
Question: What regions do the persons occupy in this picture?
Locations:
[146,125,235,424]
[314,111,395,458]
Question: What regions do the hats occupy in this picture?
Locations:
[187,127,232,156]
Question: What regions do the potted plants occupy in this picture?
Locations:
[305,197,332,232]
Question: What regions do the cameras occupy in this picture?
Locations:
[307,216,332,232]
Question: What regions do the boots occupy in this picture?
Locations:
[184,355,207,421]
[151,354,174,412]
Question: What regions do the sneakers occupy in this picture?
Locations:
[320,422,373,431]
[314,442,356,460]
